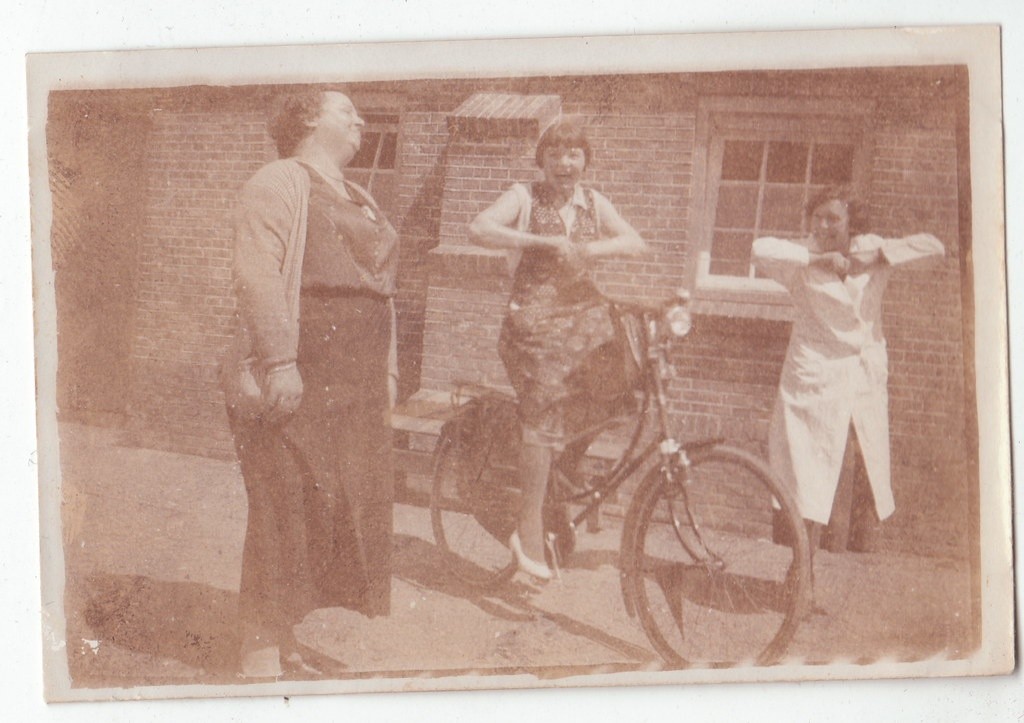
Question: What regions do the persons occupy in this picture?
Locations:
[749,184,946,610]
[219,90,400,681]
[466,117,647,579]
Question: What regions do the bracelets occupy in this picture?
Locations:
[267,361,297,374]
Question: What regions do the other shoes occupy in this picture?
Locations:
[235,641,323,683]
[782,579,826,616]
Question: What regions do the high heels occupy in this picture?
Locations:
[508,530,549,580]
[554,460,601,505]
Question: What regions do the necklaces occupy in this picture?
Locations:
[317,166,346,182]
[565,197,573,220]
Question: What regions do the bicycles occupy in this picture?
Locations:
[429,285,812,673]
